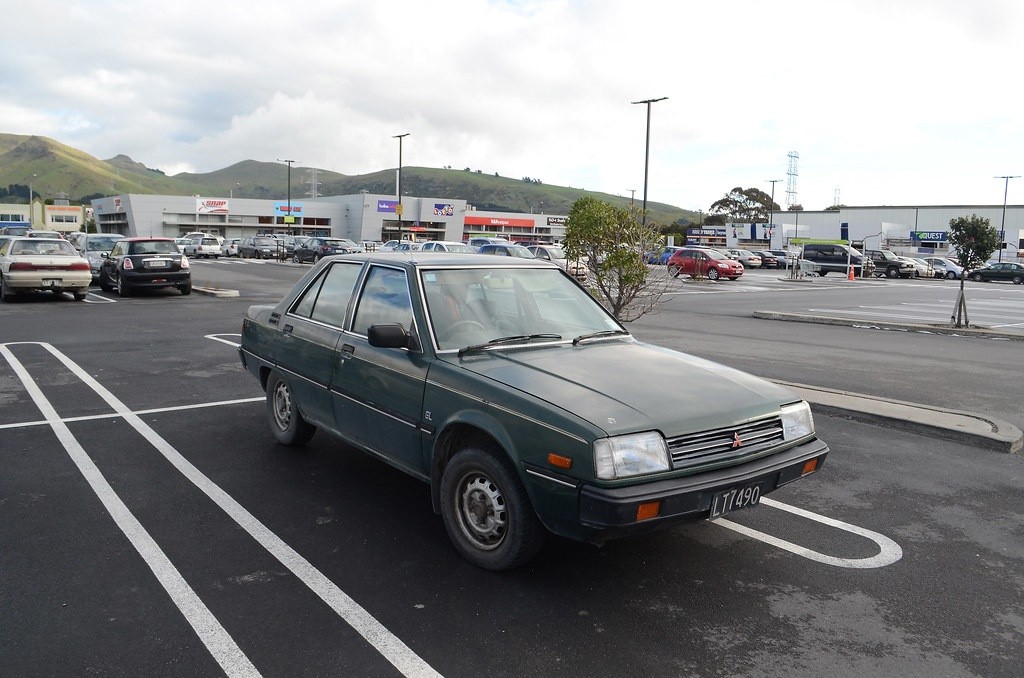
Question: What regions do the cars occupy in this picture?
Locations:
[240,252,830,574]
[175,233,589,280]
[99,237,192,297]
[898,256,994,280]
[0,226,129,301]
[556,242,799,281]
[967,262,1024,284]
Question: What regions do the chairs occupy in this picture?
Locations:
[91,242,104,251]
[135,246,145,253]
[104,241,115,251]
[154,242,170,251]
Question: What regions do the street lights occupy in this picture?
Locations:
[30,174,36,227]
[530,202,544,213]
[994,175,1022,263]
[278,159,295,236]
[765,179,784,250]
[631,96,669,264]
[231,182,240,198]
[392,133,411,244]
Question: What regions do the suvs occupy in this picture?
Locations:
[858,249,916,279]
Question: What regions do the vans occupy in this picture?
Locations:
[797,243,876,277]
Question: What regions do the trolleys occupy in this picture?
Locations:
[798,259,821,279]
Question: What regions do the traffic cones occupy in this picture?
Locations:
[849,263,856,281]
